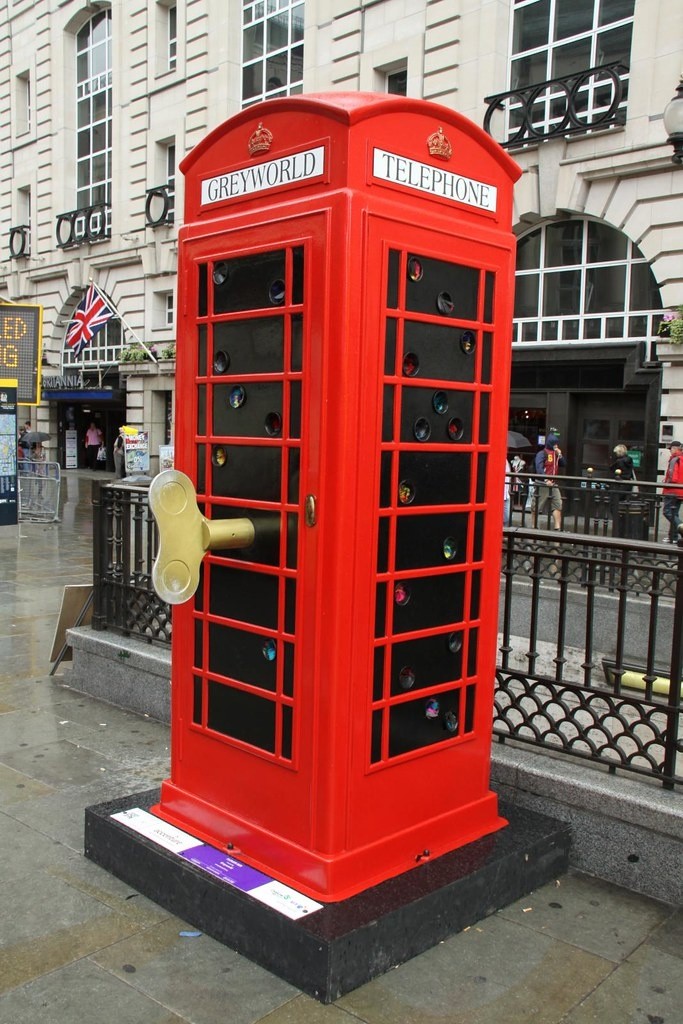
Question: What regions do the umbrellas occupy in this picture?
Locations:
[20,432,52,442]
[507,431,531,448]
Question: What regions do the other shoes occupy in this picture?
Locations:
[661,537,678,544]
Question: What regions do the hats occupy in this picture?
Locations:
[667,441,681,450]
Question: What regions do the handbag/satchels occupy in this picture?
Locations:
[630,469,639,497]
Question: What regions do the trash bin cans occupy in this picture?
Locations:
[612,500,650,542]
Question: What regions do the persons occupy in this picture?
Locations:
[30,443,49,499]
[17,421,31,476]
[511,456,525,491]
[503,459,510,542]
[609,445,633,517]
[662,441,683,543]
[113,428,124,480]
[85,422,104,471]
[531,435,567,531]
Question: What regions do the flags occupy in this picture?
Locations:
[66,284,115,359]
